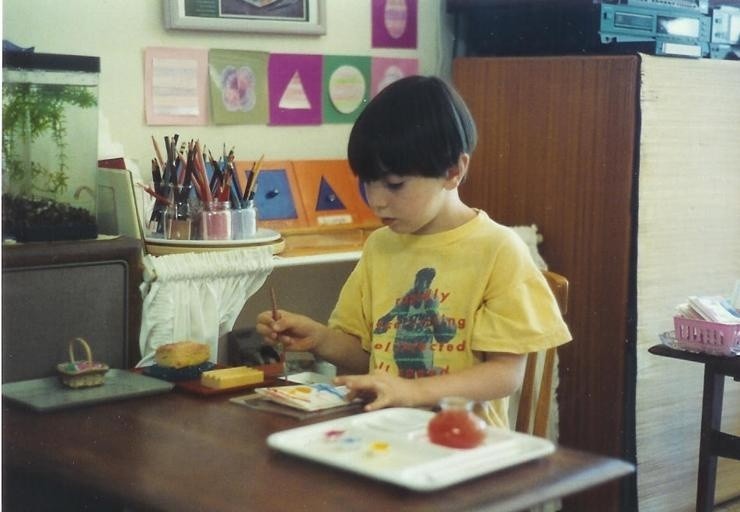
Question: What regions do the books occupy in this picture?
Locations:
[675,294,739,325]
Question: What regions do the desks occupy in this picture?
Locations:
[648,344,739,512]
[3,369,638,511]
[144,232,362,350]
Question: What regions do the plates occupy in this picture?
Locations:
[1,366,176,413]
[265,407,557,493]
[144,227,283,247]
[658,330,740,357]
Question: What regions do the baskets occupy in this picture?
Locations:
[57,337,108,388]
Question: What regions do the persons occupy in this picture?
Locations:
[377,267,455,377]
[254,75,573,434]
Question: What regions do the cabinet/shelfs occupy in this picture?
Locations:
[2,232,141,381]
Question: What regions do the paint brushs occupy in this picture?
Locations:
[137,133,266,243]
[267,285,289,387]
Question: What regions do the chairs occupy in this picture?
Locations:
[510,273,571,435]
